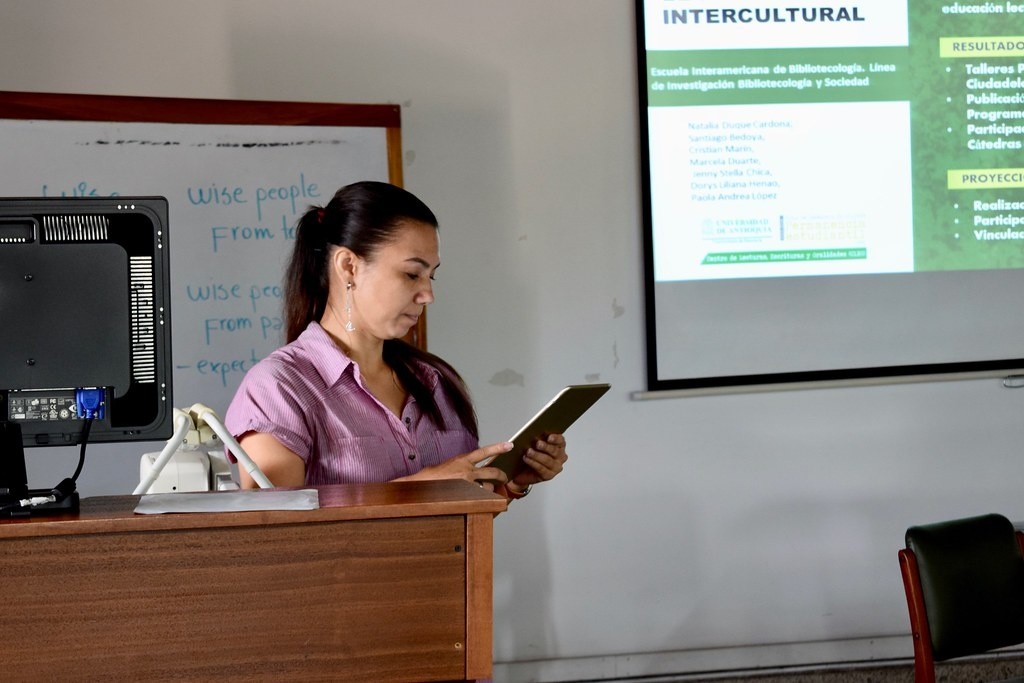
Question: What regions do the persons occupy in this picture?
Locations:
[220,182,567,518]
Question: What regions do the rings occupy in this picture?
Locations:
[477,482,484,491]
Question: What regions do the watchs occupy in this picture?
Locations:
[503,481,533,498]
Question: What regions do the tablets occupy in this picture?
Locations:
[475,386,611,492]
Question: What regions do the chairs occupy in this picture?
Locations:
[898,514,1024,683]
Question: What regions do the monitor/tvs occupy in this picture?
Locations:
[0,197,173,447]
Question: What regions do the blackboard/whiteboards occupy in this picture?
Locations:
[0,89,429,501]
